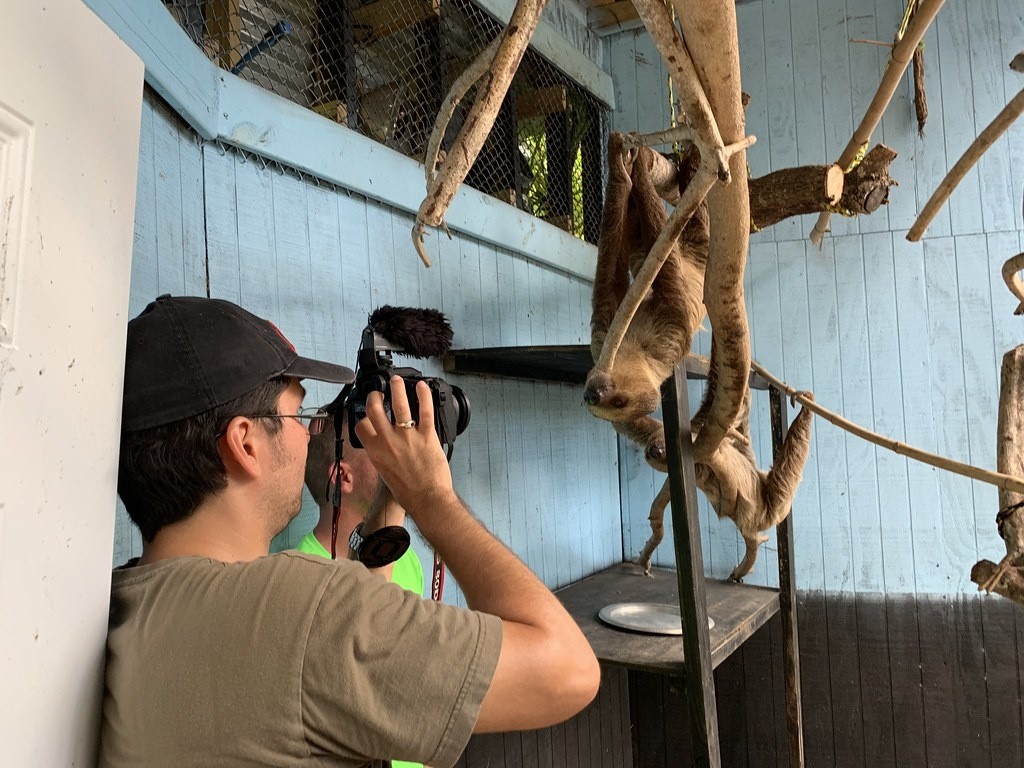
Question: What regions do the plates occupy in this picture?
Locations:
[598,602,715,635]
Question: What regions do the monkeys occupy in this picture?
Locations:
[582,131,709,424]
[611,391,814,584]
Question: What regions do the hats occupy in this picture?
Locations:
[123,294,356,431]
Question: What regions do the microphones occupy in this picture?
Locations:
[369,305,454,360]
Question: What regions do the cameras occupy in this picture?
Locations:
[349,350,472,450]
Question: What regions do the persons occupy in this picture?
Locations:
[293,401,428,768]
[93,291,602,768]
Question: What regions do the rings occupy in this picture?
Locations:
[395,421,416,428]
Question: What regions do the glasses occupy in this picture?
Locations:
[214,405,328,442]
